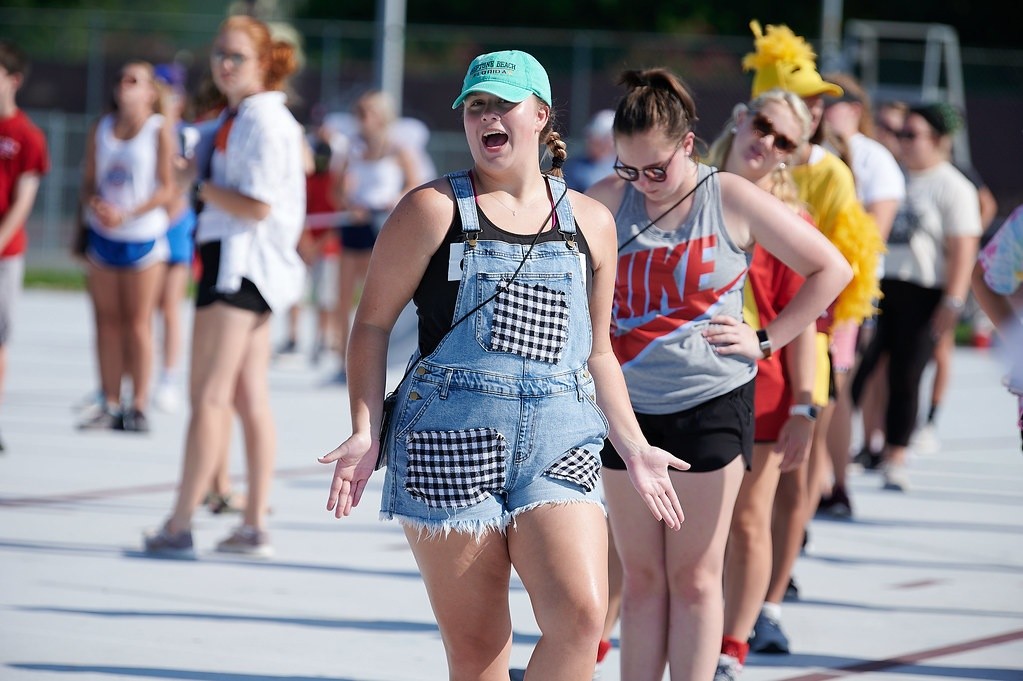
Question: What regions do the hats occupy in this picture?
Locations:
[451,50,552,111]
[741,19,844,102]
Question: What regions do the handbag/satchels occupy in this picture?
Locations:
[374,391,398,471]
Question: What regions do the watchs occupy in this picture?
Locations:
[941,293,965,315]
[788,404,818,421]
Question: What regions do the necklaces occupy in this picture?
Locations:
[475,174,538,218]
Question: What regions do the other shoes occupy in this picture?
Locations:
[781,583,797,603]
[126,519,195,561]
[712,652,744,681]
[215,525,274,556]
[811,484,851,518]
[850,448,875,468]
[751,615,789,653]
[75,396,151,431]
[204,485,249,516]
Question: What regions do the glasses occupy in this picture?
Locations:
[748,109,797,152]
[612,144,680,183]
[212,50,248,64]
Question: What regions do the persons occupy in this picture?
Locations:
[0,52,52,451]
[582,44,1023,681]
[317,50,691,681]
[85,15,438,561]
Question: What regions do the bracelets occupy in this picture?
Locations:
[756,330,771,359]
